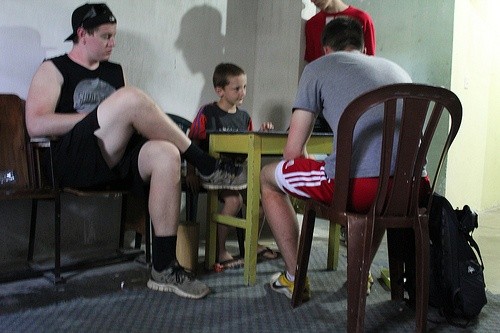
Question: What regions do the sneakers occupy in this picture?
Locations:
[195,156,248,191]
[146,259,210,299]
[366,270,374,296]
[270,271,310,300]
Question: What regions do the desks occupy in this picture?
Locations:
[205,129,343,287]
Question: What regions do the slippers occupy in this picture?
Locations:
[218,257,244,269]
[257,246,280,261]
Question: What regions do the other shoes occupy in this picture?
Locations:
[339,225,346,245]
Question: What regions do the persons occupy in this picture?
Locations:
[26,2,247,299]
[188,63,279,270]
[259,16,430,300]
[304,0,376,247]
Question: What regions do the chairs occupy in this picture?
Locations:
[290,83,461,332]
[0,93,245,306]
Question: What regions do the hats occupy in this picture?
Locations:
[64,3,115,43]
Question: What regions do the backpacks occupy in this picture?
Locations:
[404,191,488,323]
